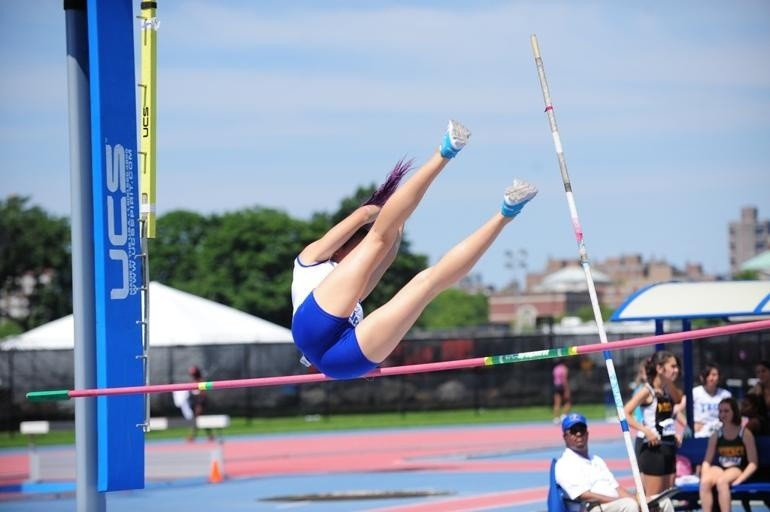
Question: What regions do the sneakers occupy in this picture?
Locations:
[439,119,473,160]
[501,175,540,217]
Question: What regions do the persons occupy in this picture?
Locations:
[626,354,650,426]
[741,361,770,444]
[287,113,541,382]
[186,366,219,445]
[737,392,761,429]
[552,407,639,512]
[613,347,689,499]
[692,395,759,512]
[676,364,740,474]
[550,361,573,424]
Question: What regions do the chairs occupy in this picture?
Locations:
[547,459,599,512]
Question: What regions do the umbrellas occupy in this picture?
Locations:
[4,278,293,352]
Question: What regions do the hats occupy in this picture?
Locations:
[561,413,588,431]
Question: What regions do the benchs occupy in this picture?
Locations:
[669,435,770,512]
